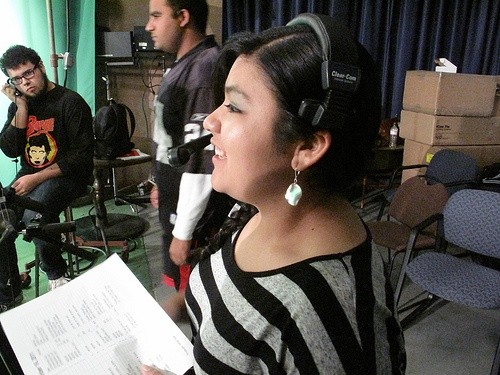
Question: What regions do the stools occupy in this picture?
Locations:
[53,184,112,282]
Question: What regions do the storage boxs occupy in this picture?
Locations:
[401,137,500,188]
[399,109,500,146]
[404,71,498,118]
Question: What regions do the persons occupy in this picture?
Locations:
[134,12,411,374]
[1,42,95,303]
[141,0,234,324]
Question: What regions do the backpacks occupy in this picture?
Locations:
[93,99,135,159]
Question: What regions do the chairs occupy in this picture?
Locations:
[377,149,482,274]
[390,189,500,375]
[361,174,451,315]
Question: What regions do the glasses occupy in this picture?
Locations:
[11,63,38,84]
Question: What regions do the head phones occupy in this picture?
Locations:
[284,13,363,134]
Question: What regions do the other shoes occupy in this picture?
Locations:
[47,275,70,291]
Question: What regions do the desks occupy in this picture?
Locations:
[67,136,160,243]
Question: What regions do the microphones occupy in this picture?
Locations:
[166,133,213,168]
